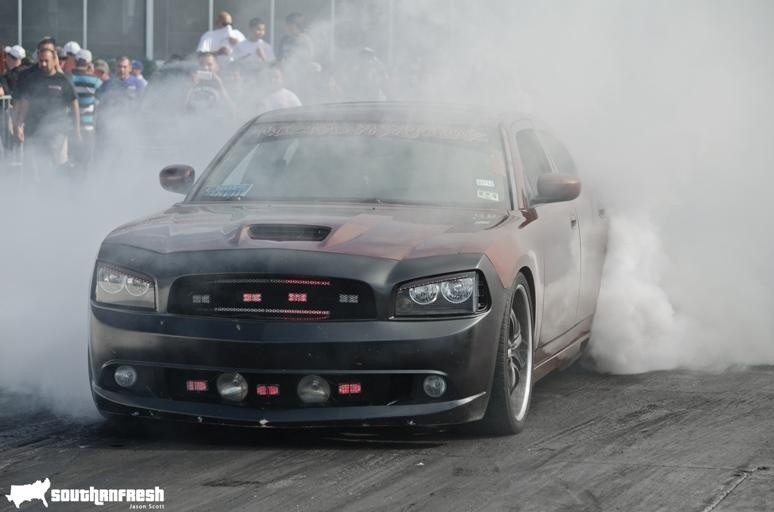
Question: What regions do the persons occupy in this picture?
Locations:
[0,12,391,174]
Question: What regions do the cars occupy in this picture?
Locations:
[86,94,614,436]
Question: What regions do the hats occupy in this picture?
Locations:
[215,12,233,25]
[57,41,109,74]
[4,45,26,60]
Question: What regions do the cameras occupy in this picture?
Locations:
[197,71,212,82]
